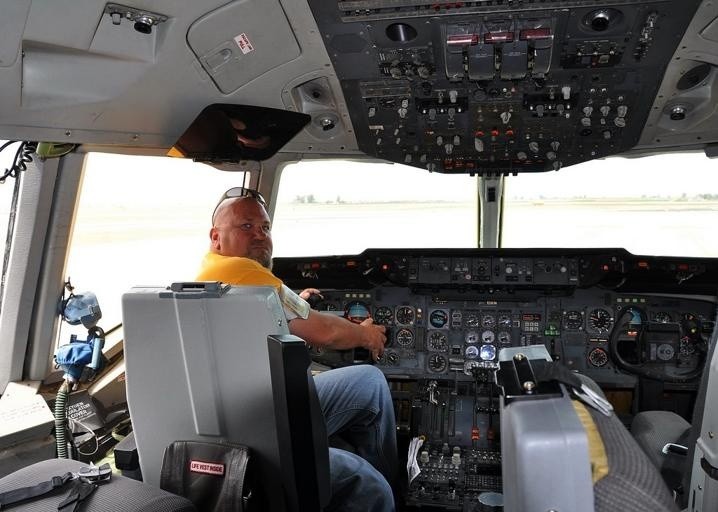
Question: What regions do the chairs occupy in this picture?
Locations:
[497,341,675,511]
[121,282,335,511]
[0,457,192,509]
[630,317,716,511]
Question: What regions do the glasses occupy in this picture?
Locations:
[212,187,268,217]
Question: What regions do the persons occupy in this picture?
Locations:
[191,193,406,511]
